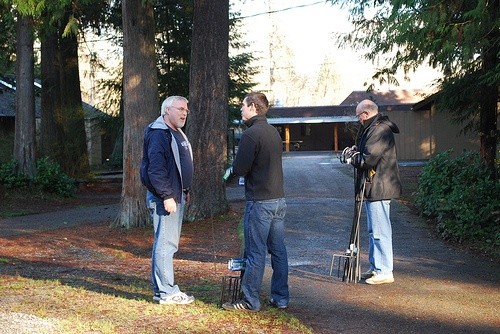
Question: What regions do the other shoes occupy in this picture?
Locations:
[361,268,394,284]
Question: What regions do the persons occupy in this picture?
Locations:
[340,98,403,284]
[141,95,195,305]
[221,91,291,311]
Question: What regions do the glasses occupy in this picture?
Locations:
[171,106,189,113]
[356,111,366,119]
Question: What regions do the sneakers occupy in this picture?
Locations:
[152,285,194,304]
[268,297,287,308]
[221,298,260,312]
